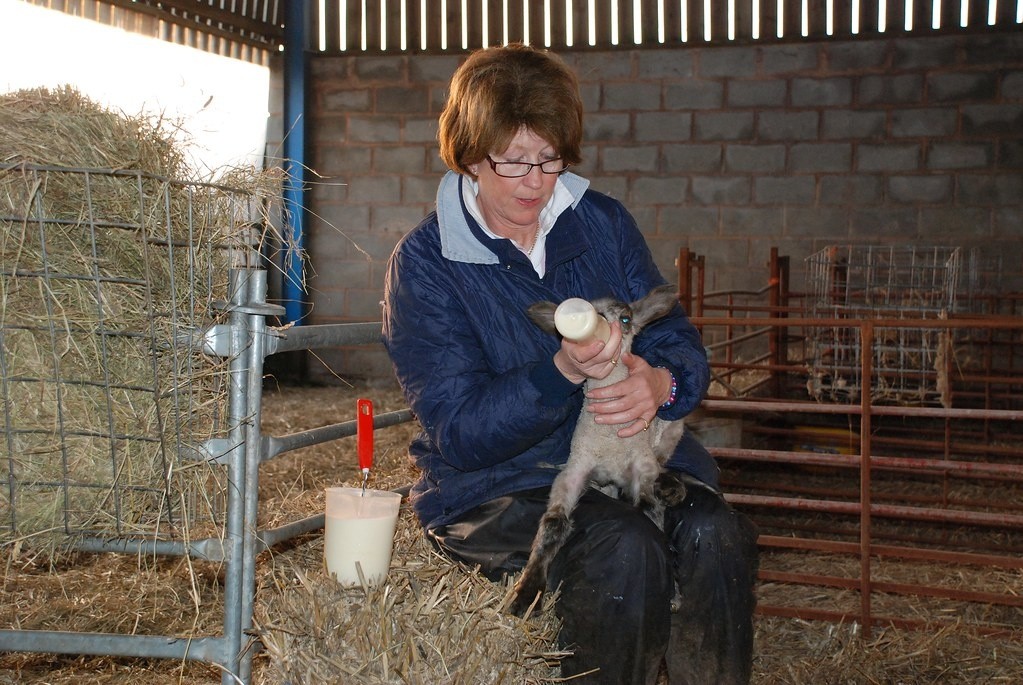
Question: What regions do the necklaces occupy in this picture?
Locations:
[528,221,540,256]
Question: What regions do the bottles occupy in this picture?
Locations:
[556,298,611,345]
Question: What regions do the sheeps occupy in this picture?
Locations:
[495,283,685,618]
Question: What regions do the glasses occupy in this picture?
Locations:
[485,152,570,177]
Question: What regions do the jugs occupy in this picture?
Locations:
[323,488,402,588]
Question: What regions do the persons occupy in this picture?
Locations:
[380,46,758,685]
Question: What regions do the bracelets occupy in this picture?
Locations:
[657,365,676,408]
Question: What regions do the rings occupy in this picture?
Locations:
[610,357,617,365]
[639,418,649,431]
